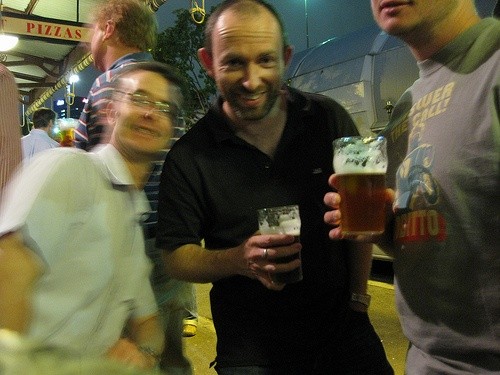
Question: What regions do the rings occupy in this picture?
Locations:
[263,248,268,260]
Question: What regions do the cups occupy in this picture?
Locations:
[332,137,388,235]
[259,206,304,289]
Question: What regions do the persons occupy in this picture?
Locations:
[182,283,198,337]
[57,0,197,375]
[156,0,396,375]
[20,108,64,165]
[0,61,188,375]
[323,0,500,375]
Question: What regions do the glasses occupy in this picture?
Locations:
[115,89,176,120]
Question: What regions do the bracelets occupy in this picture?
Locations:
[138,345,161,360]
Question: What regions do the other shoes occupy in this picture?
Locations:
[182,319,198,336]
